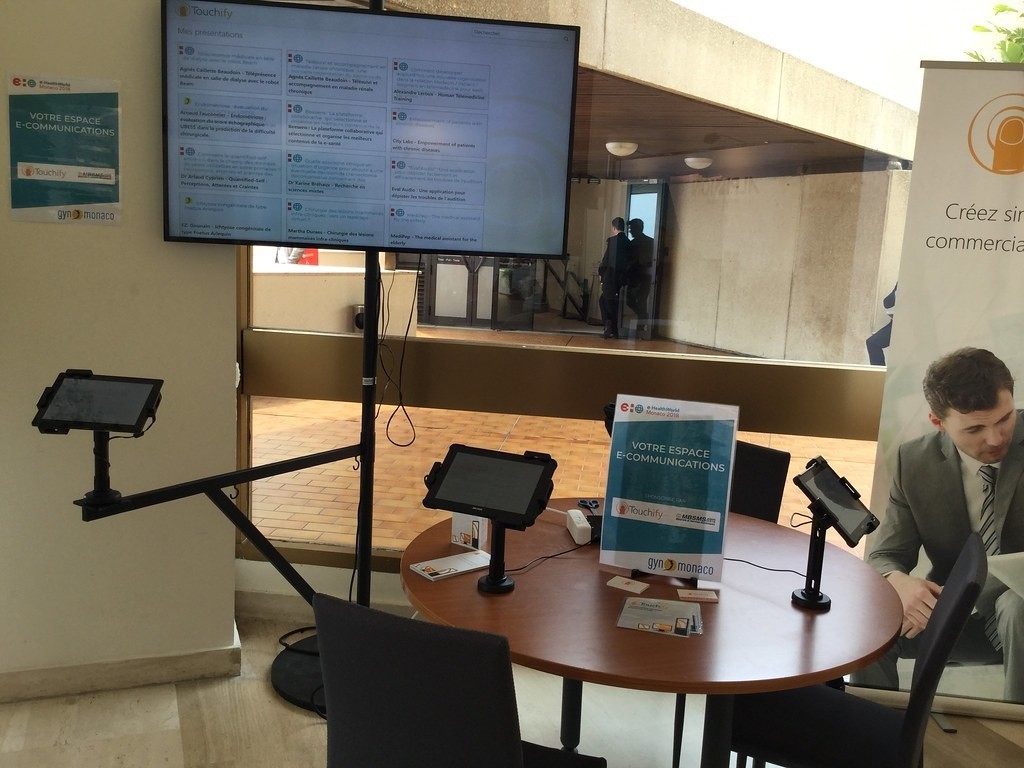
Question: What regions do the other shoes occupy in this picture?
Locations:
[601,332,619,340]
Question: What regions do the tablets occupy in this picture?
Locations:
[32,372,165,433]
[794,458,873,548]
[423,444,558,527]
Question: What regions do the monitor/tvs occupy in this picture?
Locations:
[160,0,581,260]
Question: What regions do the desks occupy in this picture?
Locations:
[400,497,904,768]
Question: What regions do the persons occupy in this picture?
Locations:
[626,219,654,340]
[598,217,632,339]
[848,346,1024,705]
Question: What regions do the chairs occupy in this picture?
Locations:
[728,439,791,525]
[312,592,607,768]
[730,531,988,768]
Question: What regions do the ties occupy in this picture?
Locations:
[977,466,1003,652]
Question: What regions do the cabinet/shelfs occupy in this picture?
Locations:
[251,264,423,337]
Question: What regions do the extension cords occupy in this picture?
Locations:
[567,510,592,545]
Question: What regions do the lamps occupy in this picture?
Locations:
[605,142,638,157]
[684,158,713,170]
